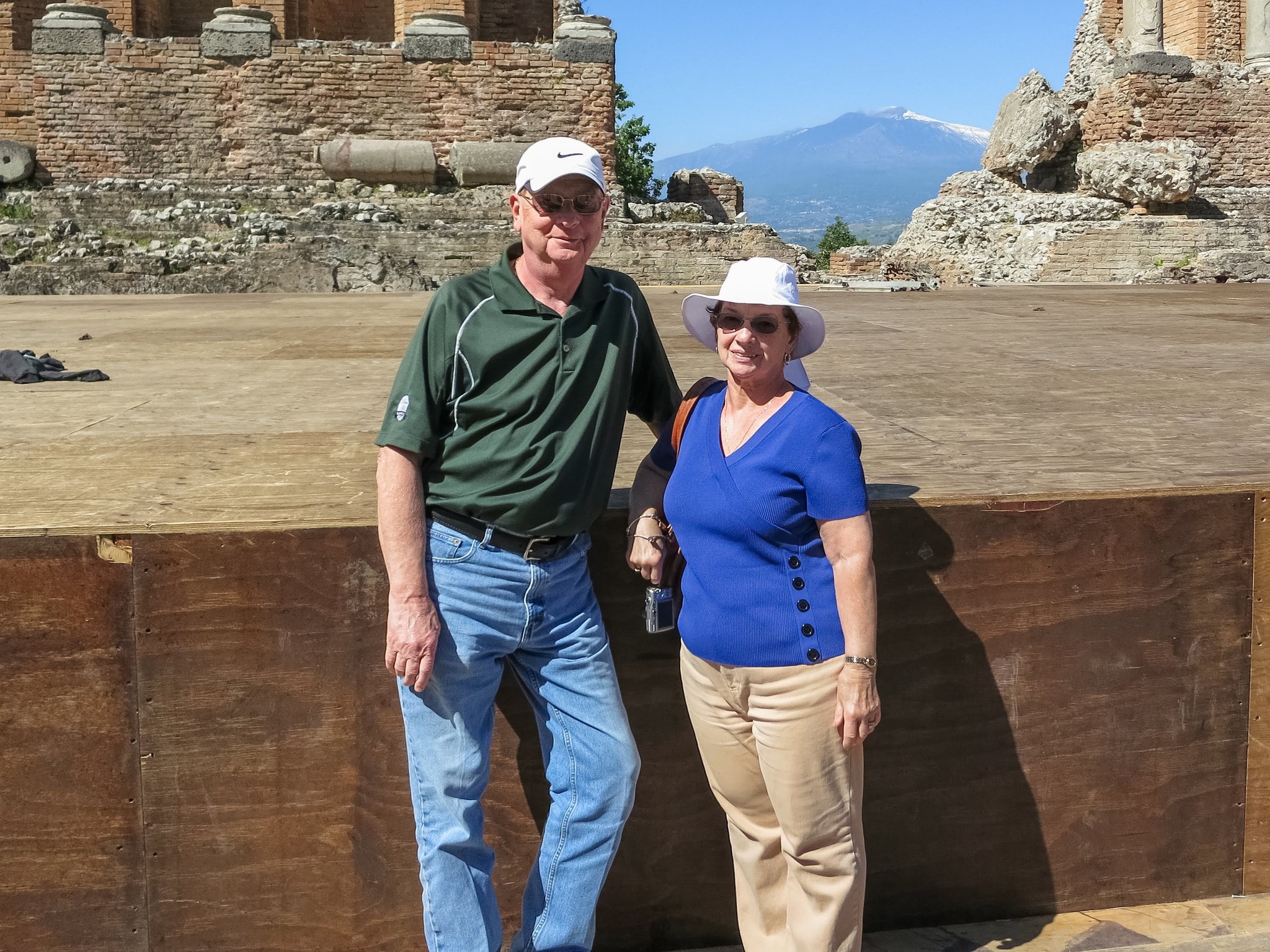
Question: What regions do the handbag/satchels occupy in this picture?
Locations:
[653,377,719,631]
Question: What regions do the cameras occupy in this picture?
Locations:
[645,586,676,633]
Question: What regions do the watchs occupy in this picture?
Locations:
[845,655,878,668]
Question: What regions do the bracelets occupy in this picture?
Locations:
[627,513,681,555]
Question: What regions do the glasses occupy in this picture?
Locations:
[518,194,605,214]
[716,313,790,335]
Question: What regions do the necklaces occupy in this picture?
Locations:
[723,388,777,454]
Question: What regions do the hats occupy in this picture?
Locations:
[681,256,824,360]
[515,137,605,197]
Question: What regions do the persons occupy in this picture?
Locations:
[376,138,682,952]
[617,257,882,952]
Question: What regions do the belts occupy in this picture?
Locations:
[425,504,574,562]
